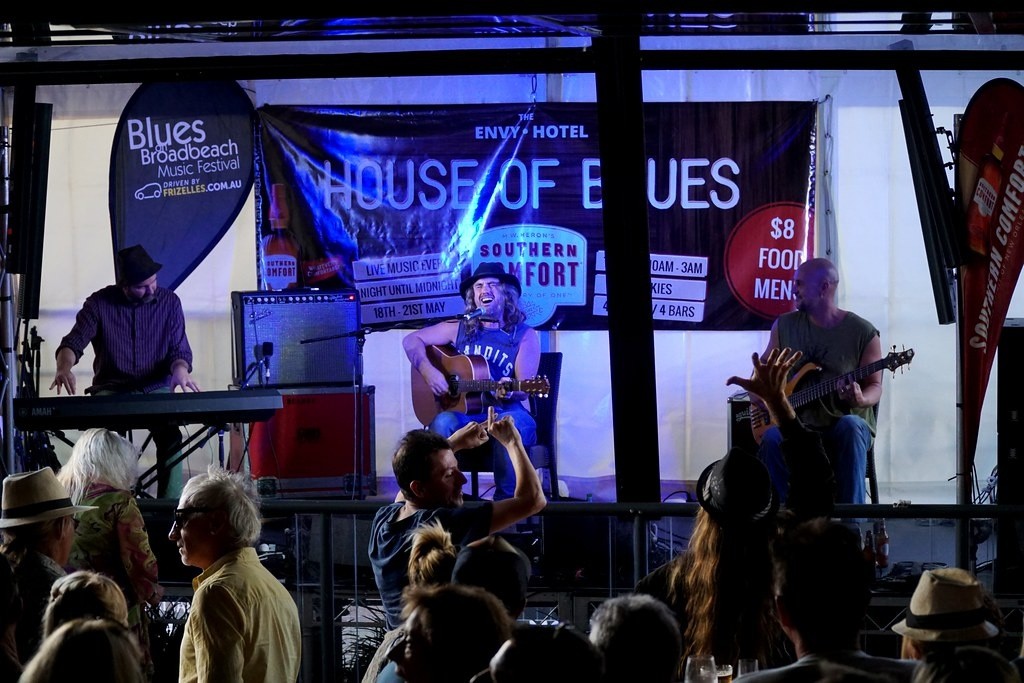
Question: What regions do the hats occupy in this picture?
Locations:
[696,447,772,528]
[0,466,100,529]
[116,244,163,290]
[890,567,999,642]
[450,534,532,609]
[459,262,523,302]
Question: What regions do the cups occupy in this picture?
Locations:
[718,664,733,683]
[737,659,758,677]
[683,654,718,683]
[257,477,280,501]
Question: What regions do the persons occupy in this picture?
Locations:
[168,466,301,683]
[748,258,882,522]
[49,244,201,500]
[19,571,144,683]
[633,347,876,683]
[361,522,1024,683]
[54,427,164,683]
[402,261,541,503]
[368,406,547,636]
[0,467,100,683]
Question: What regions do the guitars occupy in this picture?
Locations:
[409,343,553,426]
[749,346,919,444]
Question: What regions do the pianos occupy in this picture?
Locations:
[0,386,288,498]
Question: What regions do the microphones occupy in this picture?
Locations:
[262,342,273,380]
[464,307,487,320]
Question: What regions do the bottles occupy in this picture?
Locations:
[291,215,347,290]
[863,518,890,569]
[259,180,298,290]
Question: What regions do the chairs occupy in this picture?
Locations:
[454,352,563,498]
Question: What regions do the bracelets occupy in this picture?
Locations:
[147,584,158,603]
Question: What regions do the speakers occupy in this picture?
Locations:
[232,289,364,388]
[284,511,375,591]
[5,52,38,273]
[887,39,970,268]
[897,98,957,324]
[995,318,1024,598]
[542,510,649,591]
[16,103,53,319]
[135,498,202,587]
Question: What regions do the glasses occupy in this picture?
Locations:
[173,507,218,522]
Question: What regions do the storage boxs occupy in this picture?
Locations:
[228,384,379,499]
